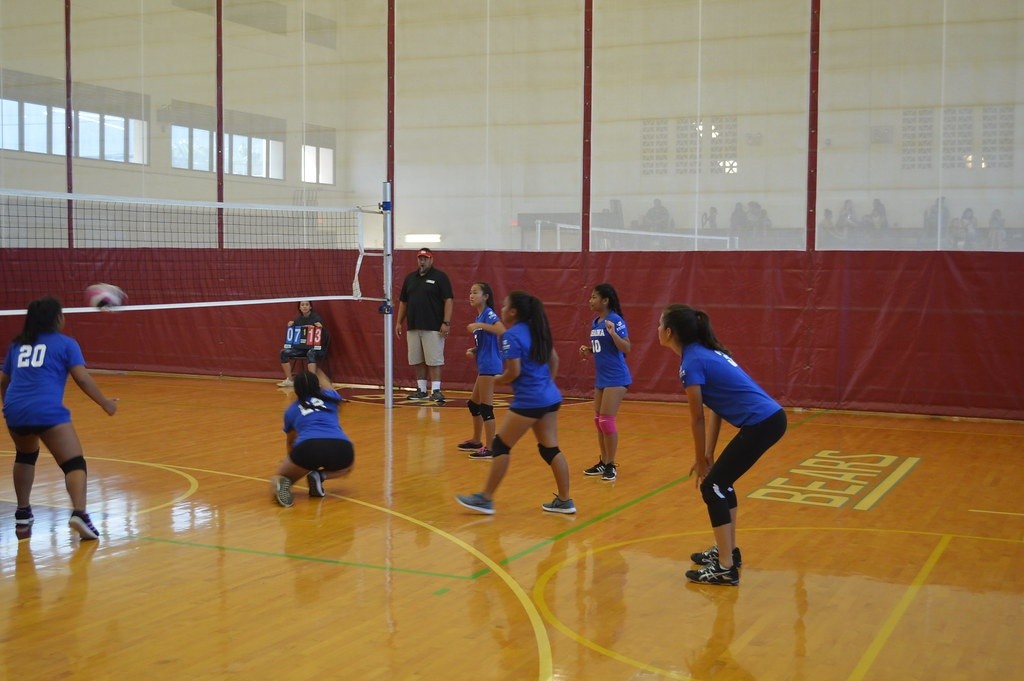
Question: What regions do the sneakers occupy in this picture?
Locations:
[468,446,494,459]
[407,388,430,400]
[601,461,620,480]
[277,377,294,387]
[14,505,34,525]
[690,545,742,566]
[542,492,576,514]
[458,439,483,452]
[455,491,496,515]
[69,509,100,540]
[271,473,295,507]
[685,558,740,585]
[583,455,607,475]
[431,388,446,402]
[307,470,326,497]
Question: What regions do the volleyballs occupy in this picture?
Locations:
[85,284,123,308]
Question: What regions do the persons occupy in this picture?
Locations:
[277,300,328,387]
[658,304,786,586]
[579,283,633,481]
[0,298,117,540]
[455,291,577,515]
[457,282,506,459]
[271,367,354,509]
[395,248,454,401]
[648,198,1007,250]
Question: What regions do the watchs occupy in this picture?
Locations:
[443,321,450,326]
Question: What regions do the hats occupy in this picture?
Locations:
[417,249,433,258]
